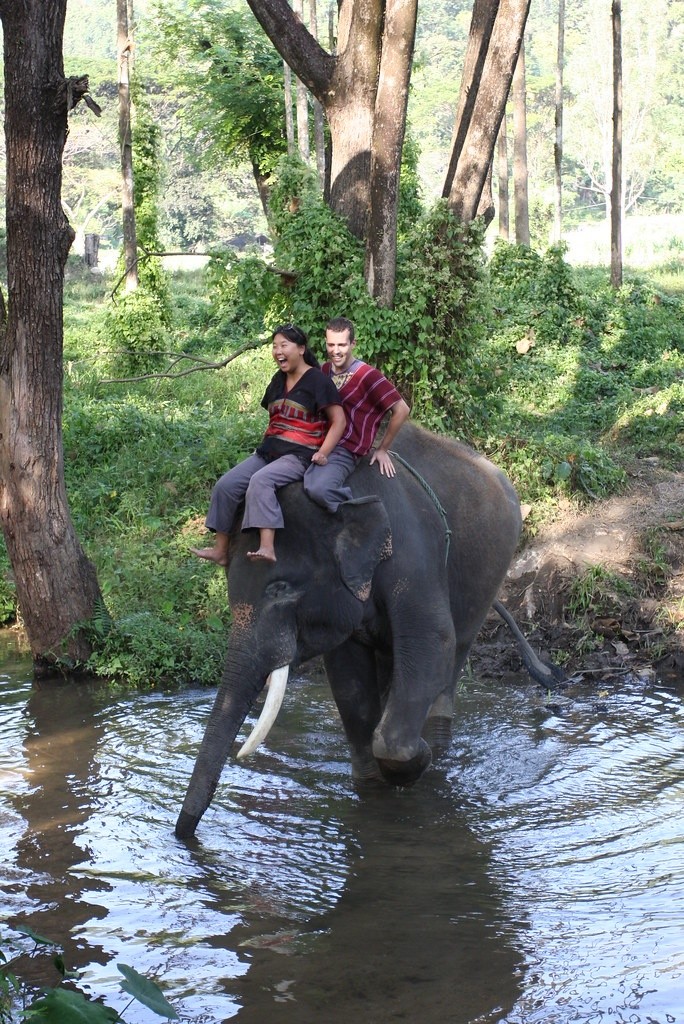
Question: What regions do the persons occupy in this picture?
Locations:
[307,320,411,508]
[186,325,347,564]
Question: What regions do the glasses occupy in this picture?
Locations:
[277,323,304,340]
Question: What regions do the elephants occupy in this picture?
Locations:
[175,421,552,838]
